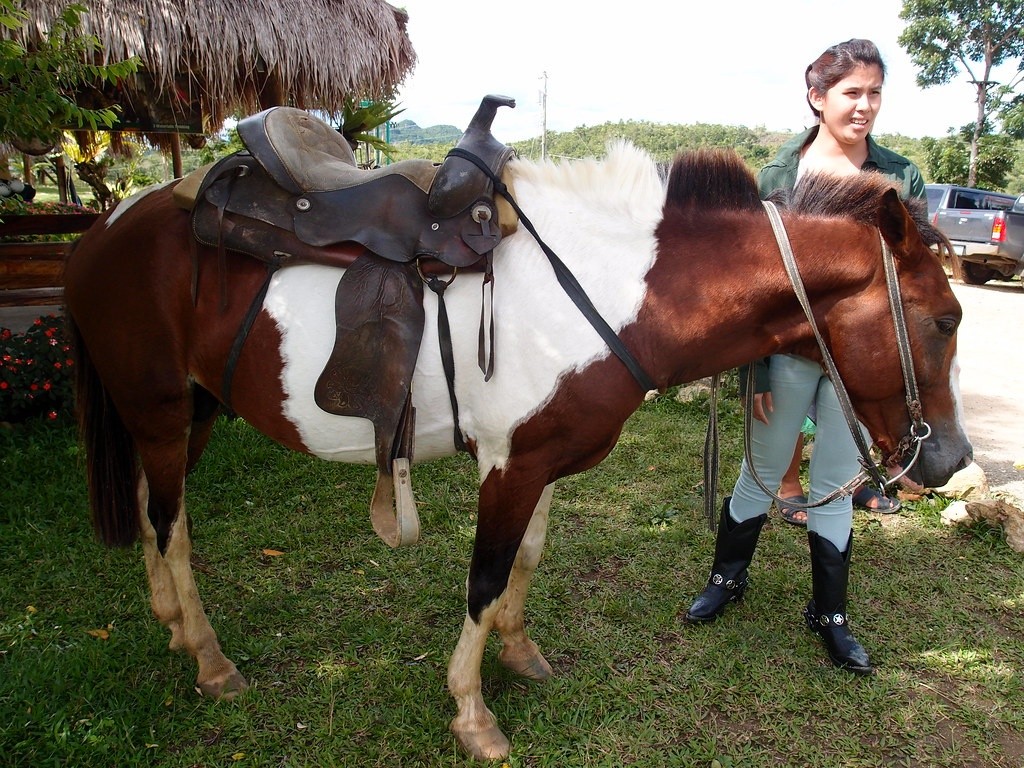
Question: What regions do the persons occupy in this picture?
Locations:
[777,416,902,525]
[684,37,928,674]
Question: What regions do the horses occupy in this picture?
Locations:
[60,150,977,760]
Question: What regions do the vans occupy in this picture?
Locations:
[924,183,1018,257]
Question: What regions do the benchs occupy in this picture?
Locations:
[0,213,100,307]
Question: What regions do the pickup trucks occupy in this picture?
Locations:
[929,193,1024,286]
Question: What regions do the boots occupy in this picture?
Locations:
[804,529,874,672]
[686,496,768,623]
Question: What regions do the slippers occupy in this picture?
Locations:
[774,490,807,525]
[853,484,902,515]
